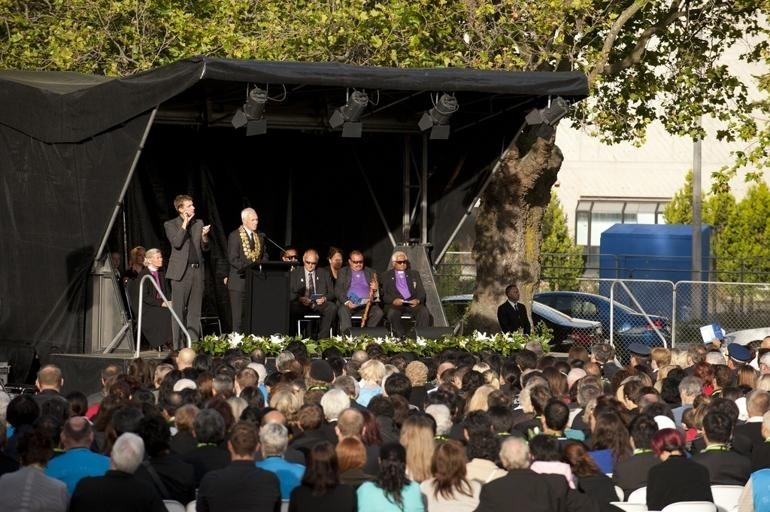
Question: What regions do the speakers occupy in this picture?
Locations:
[345,326,390,339]
[411,327,454,339]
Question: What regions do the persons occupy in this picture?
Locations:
[335,250,383,336]
[381,252,430,338]
[1,343,769,512]
[163,195,212,350]
[128,248,172,352]
[283,247,298,273]
[499,284,531,338]
[288,248,337,339]
[323,249,345,287]
[112,250,127,319]
[124,246,146,281]
[226,207,267,334]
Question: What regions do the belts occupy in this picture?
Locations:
[186,263,201,268]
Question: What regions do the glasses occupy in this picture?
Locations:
[306,261,318,266]
[284,255,298,261]
[393,261,407,265]
[350,258,364,264]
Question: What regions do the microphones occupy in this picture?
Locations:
[258,232,286,253]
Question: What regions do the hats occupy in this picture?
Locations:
[629,343,653,359]
[726,342,752,364]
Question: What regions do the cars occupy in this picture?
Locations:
[438,293,602,353]
[535,288,670,359]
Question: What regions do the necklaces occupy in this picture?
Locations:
[239,225,261,262]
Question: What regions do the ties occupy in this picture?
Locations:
[309,274,315,309]
[514,304,520,316]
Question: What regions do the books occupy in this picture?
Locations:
[348,292,371,306]
[402,300,413,304]
[309,293,323,304]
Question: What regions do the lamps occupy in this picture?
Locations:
[230,82,571,141]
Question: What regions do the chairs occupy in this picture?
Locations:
[123,282,417,352]
